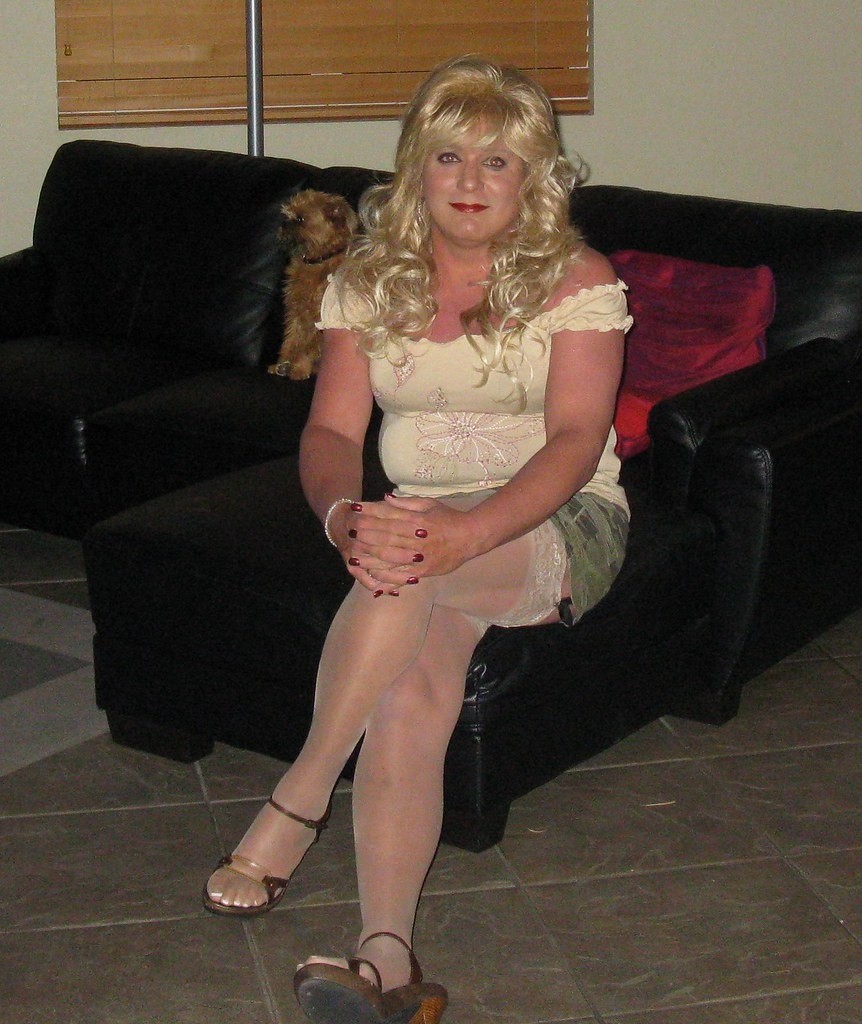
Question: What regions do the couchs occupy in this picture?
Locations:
[84,185,862,854]
[0,140,319,540]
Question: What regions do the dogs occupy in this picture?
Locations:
[265,188,360,380]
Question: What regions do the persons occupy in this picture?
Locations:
[200,52,632,1024]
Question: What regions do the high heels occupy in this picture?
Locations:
[293,932,448,1024]
[202,795,333,917]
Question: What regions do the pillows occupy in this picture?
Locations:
[602,249,778,461]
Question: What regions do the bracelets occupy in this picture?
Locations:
[323,498,355,549]
